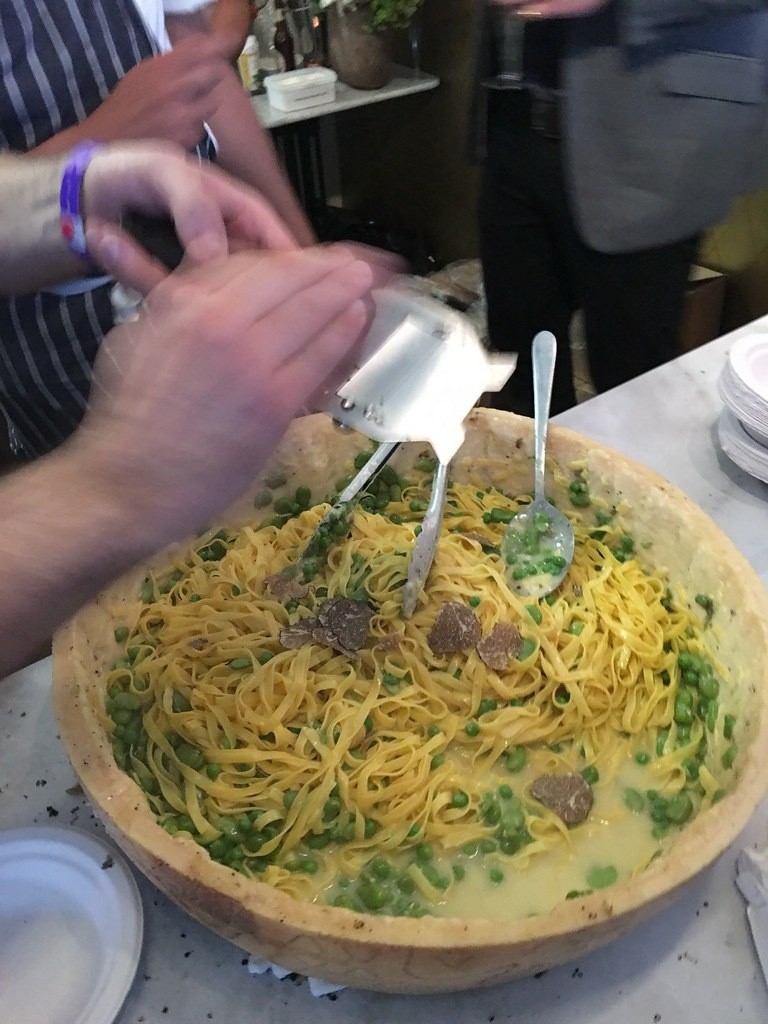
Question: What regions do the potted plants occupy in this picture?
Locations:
[328,0,425,91]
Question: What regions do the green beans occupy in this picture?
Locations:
[108,450,734,914]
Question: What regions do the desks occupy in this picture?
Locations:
[0,312,768,1024]
[252,65,440,243]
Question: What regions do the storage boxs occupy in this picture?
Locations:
[263,67,337,111]
[682,264,727,353]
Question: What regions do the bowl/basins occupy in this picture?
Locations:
[264,67,337,110]
[54,408,768,997]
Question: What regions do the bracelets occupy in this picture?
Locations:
[60,138,107,257]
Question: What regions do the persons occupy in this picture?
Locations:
[0,0,403,682]
[472,0,768,419]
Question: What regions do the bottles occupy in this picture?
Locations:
[236,34,262,92]
[273,0,296,72]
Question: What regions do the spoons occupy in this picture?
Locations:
[504,330,573,598]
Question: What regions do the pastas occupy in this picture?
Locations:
[88,479,721,877]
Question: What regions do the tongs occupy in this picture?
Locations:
[296,441,450,622]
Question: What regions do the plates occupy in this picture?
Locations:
[717,335,768,486]
[0,823,145,1024]
